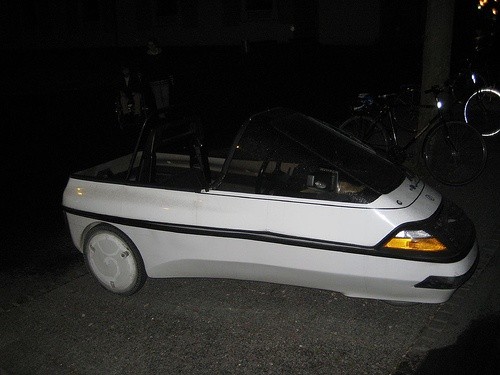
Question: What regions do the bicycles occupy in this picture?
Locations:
[337,92,489,185]
[396,53,500,138]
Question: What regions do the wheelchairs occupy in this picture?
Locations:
[111,96,152,136]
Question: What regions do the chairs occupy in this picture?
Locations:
[126,105,218,193]
